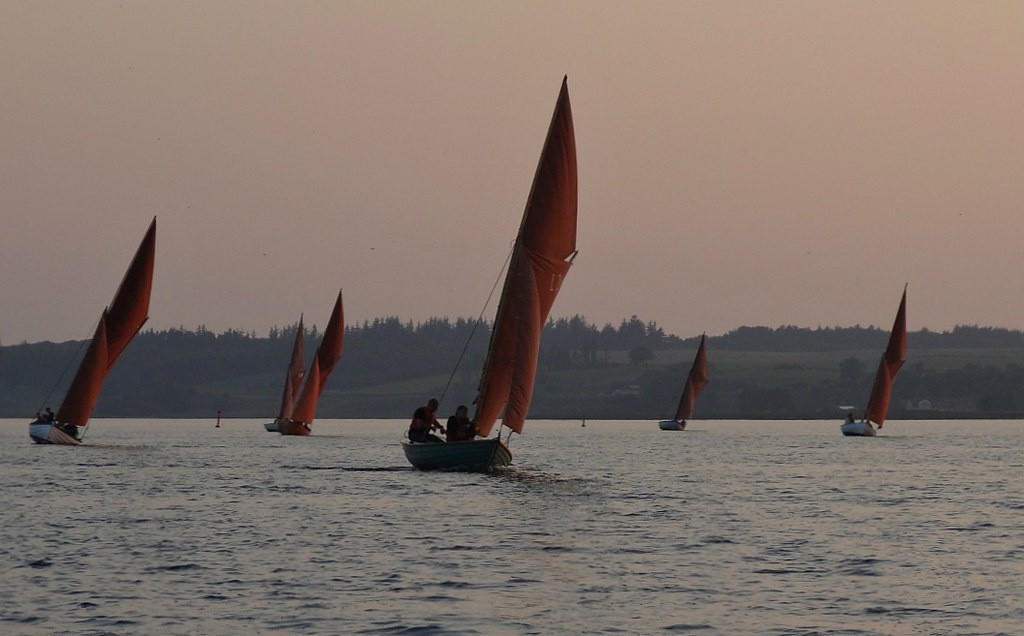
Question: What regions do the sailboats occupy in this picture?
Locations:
[29,214,159,447]
[840,284,908,439]
[655,329,712,431]
[396,75,584,472]
[264,310,306,432]
[277,286,348,440]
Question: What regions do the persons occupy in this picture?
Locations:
[409,399,445,442]
[63,417,79,435]
[36,407,54,425]
[446,405,474,442]
